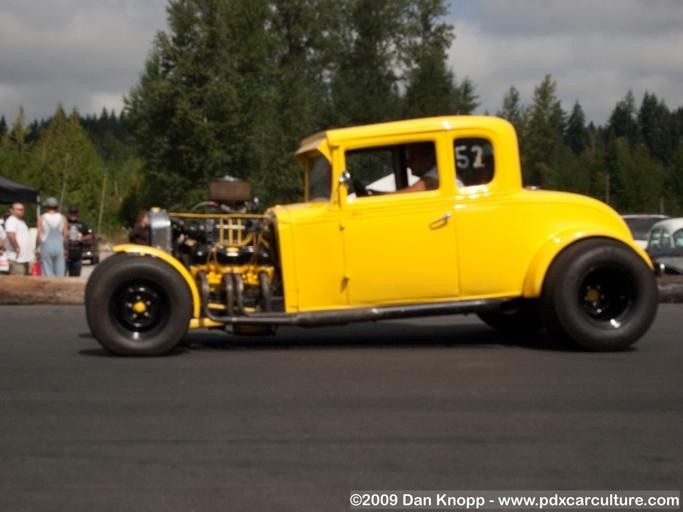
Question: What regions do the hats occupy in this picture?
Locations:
[42,197,58,207]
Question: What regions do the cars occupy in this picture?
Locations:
[86,115,658,356]
[622,214,683,276]
[81,229,101,265]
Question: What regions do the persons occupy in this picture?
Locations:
[128,208,148,246]
[0,196,92,277]
[366,142,465,195]
